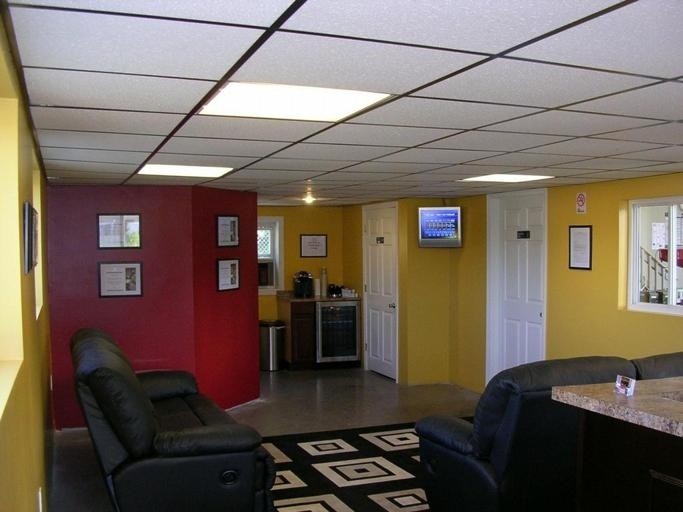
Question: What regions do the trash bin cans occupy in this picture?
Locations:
[260,320,286,371]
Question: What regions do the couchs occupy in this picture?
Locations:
[69,328,278,511]
[414,347,683,506]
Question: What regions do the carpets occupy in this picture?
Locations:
[261,415,474,511]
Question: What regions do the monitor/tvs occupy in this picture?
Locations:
[418,206,462,248]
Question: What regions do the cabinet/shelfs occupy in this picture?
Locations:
[278,293,363,368]
[96,260,143,298]
[95,212,141,250]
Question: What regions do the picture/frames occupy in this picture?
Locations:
[298,232,327,257]
[568,224,592,270]
[215,257,241,291]
[215,213,239,249]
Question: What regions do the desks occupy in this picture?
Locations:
[550,376,683,511]
